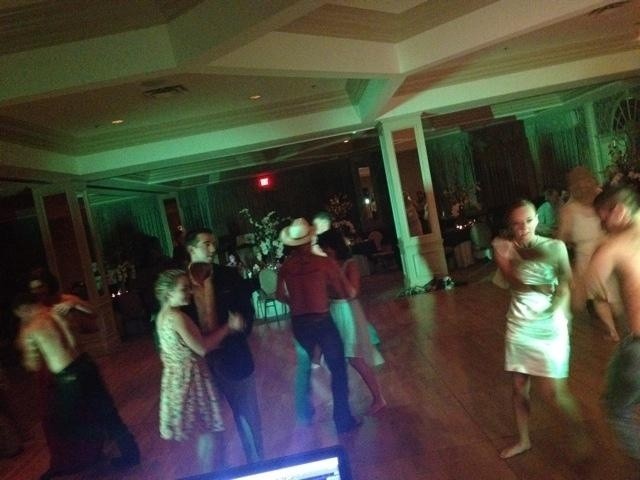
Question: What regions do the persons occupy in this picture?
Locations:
[112,209,334,286]
[276,216,367,433]
[172,227,266,466]
[27,271,108,472]
[492,199,584,458]
[537,165,639,463]
[152,266,248,473]
[402,187,433,237]
[316,226,388,417]
[10,292,143,479]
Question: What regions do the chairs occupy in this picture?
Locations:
[470,224,494,260]
[257,269,287,321]
[367,231,383,251]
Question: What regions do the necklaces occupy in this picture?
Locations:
[512,237,537,249]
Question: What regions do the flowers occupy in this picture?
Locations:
[105,261,136,286]
[243,206,291,266]
[334,219,357,234]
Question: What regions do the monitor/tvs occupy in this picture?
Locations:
[175,444,354,480]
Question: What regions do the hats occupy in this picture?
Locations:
[280,218,316,246]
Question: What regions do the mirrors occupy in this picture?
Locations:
[42,192,104,305]
[390,127,433,237]
